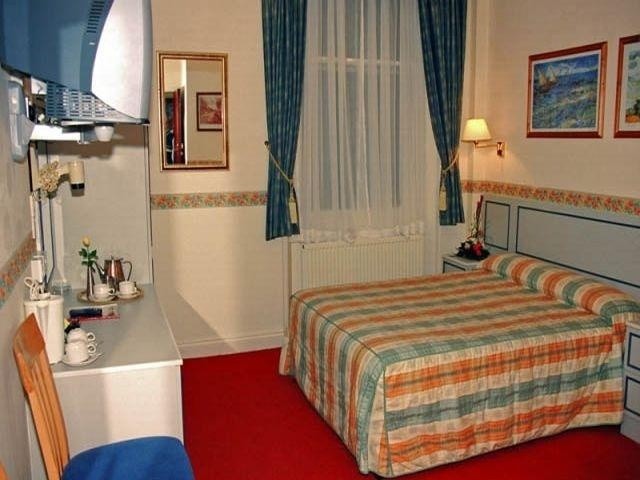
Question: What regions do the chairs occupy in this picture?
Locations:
[7,312,197,480]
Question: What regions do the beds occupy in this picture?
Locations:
[280,249,639,478]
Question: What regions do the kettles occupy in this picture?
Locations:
[24,293,65,364]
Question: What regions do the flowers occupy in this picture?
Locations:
[78,235,98,268]
[446,192,493,259]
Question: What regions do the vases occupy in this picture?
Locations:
[86,265,96,299]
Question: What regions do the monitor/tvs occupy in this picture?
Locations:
[1,0,155,131]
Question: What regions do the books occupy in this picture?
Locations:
[68,302,121,323]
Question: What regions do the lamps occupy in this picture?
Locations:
[25,143,86,196]
[461,116,507,159]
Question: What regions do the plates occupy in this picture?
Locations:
[90,296,115,302]
[115,291,140,300]
[61,353,98,365]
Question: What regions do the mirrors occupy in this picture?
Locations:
[155,49,230,173]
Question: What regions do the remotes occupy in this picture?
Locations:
[69,307,103,317]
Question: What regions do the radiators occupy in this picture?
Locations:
[288,233,426,294]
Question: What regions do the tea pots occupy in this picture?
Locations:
[96,256,133,292]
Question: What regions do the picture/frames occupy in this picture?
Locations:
[612,31,640,141]
[195,88,223,133]
[523,38,607,141]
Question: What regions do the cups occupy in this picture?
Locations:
[66,341,94,362]
[120,280,136,294]
[67,328,96,343]
[94,284,114,297]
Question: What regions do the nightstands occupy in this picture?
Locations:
[617,313,640,443]
[439,250,490,274]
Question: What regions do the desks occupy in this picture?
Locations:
[20,281,188,480]
